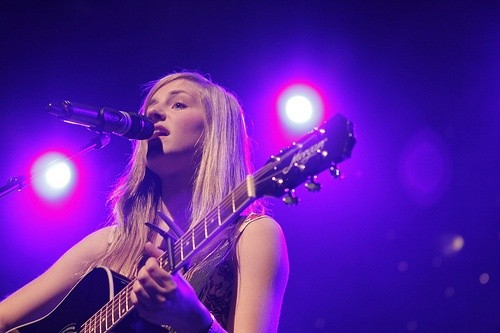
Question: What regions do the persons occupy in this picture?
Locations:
[0,69,289,333]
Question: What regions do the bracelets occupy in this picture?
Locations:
[205,310,219,333]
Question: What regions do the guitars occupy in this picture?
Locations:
[5,112,357,332]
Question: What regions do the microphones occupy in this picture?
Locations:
[45,99,154,140]
